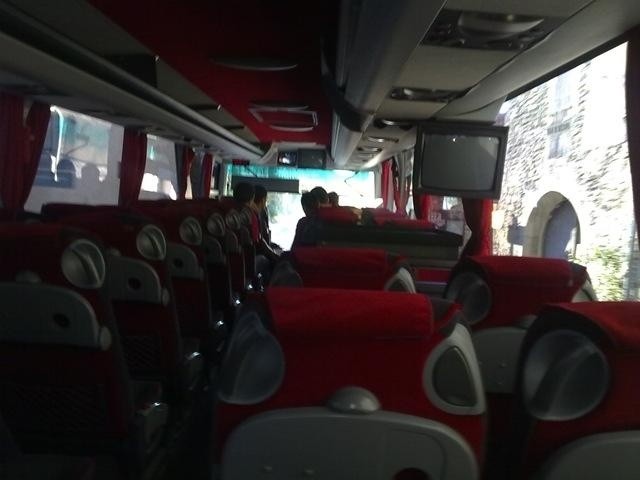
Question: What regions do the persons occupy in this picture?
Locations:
[311,188,330,208]
[255,184,270,246]
[327,192,342,209]
[233,181,281,279]
[291,194,319,251]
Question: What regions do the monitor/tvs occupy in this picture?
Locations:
[412,121,509,200]
[298,149,326,169]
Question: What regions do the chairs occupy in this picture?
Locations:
[0,194,640,480]
